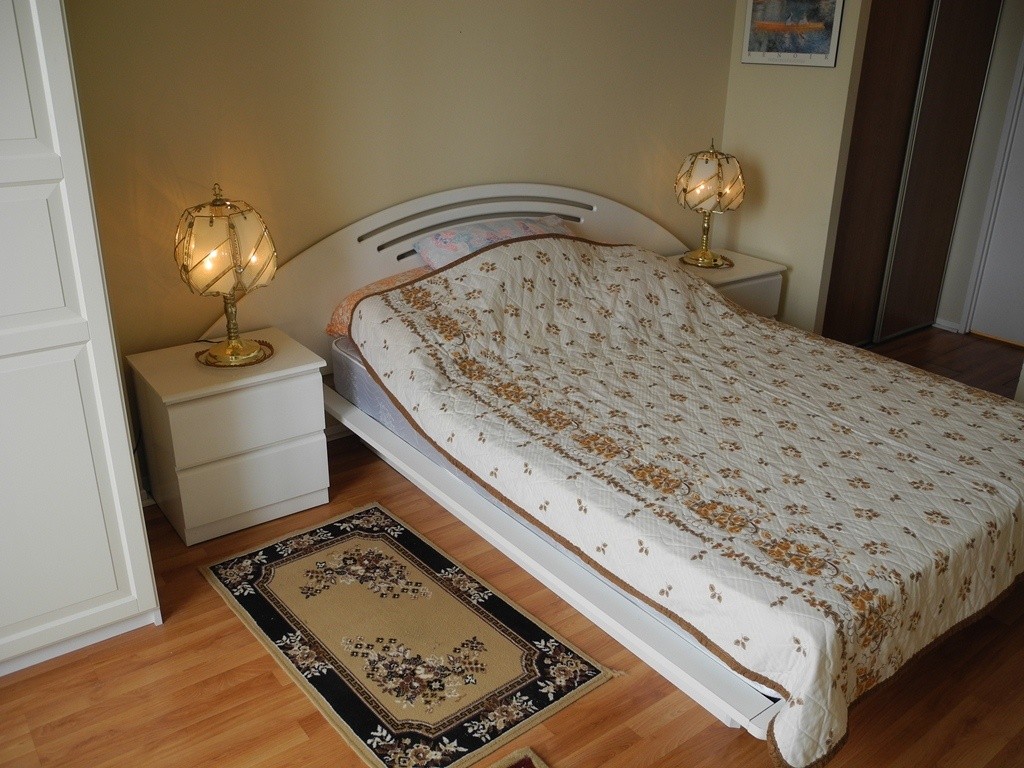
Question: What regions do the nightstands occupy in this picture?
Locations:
[667,248,788,321]
[125,326,330,547]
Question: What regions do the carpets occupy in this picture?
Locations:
[490,747,551,768]
[197,499,620,768]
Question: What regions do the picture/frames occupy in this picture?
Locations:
[741,1,845,67]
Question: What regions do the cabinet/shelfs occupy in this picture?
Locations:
[2,0,166,674]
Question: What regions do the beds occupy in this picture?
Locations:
[201,182,1024,768]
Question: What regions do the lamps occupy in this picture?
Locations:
[173,182,280,370]
[675,137,745,270]
